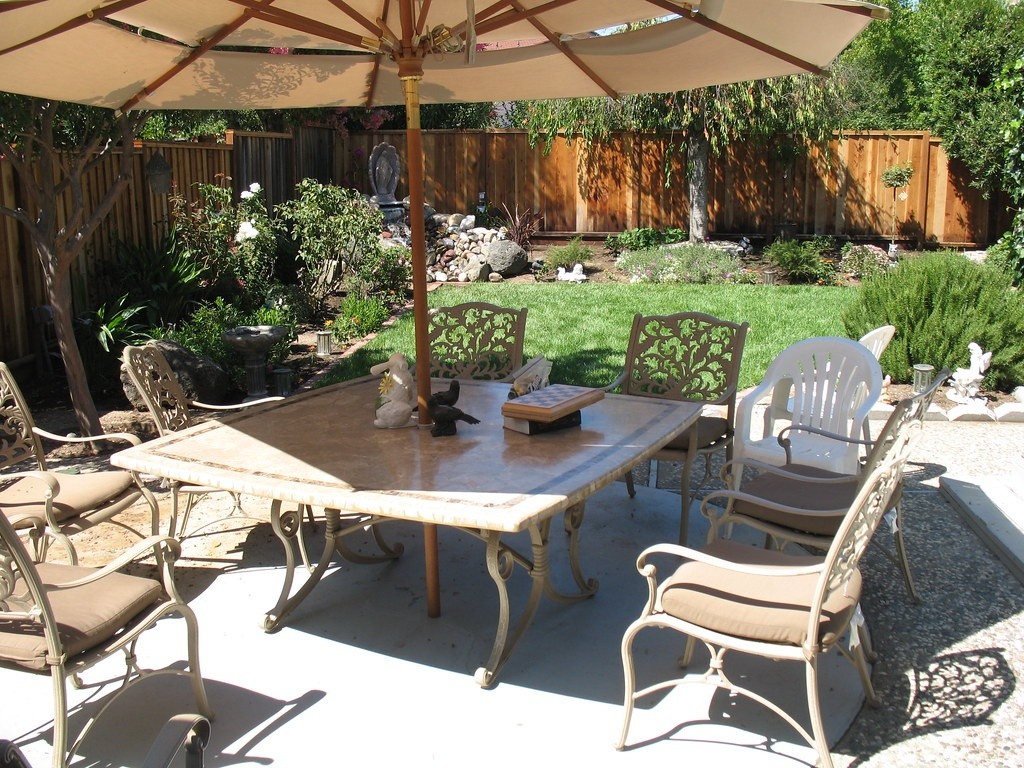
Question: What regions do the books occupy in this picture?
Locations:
[500,384,605,436]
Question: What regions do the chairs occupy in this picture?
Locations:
[428,301,554,385]
[0,340,317,767]
[592,312,749,506]
[704,374,951,659]
[613,417,921,768]
[728,337,884,476]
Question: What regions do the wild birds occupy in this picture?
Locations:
[411,379,481,436]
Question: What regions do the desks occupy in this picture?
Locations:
[109,372,704,689]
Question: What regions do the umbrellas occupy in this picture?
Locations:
[0,0,895,621]
